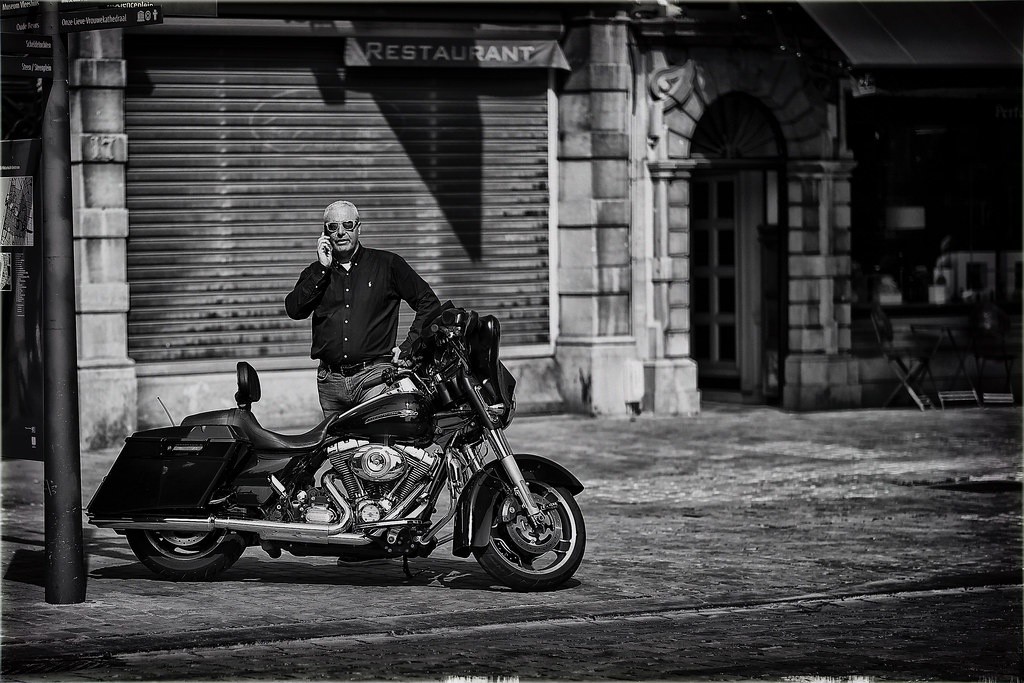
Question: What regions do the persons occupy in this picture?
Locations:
[284,201,441,567]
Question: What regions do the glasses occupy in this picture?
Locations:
[324,220,359,232]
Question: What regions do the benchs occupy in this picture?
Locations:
[869,303,1024,413]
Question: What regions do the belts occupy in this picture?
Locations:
[320,352,394,377]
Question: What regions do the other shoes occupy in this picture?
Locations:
[336,556,392,566]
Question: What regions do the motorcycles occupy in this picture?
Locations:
[84,299,587,593]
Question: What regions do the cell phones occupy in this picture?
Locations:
[323,226,330,254]
